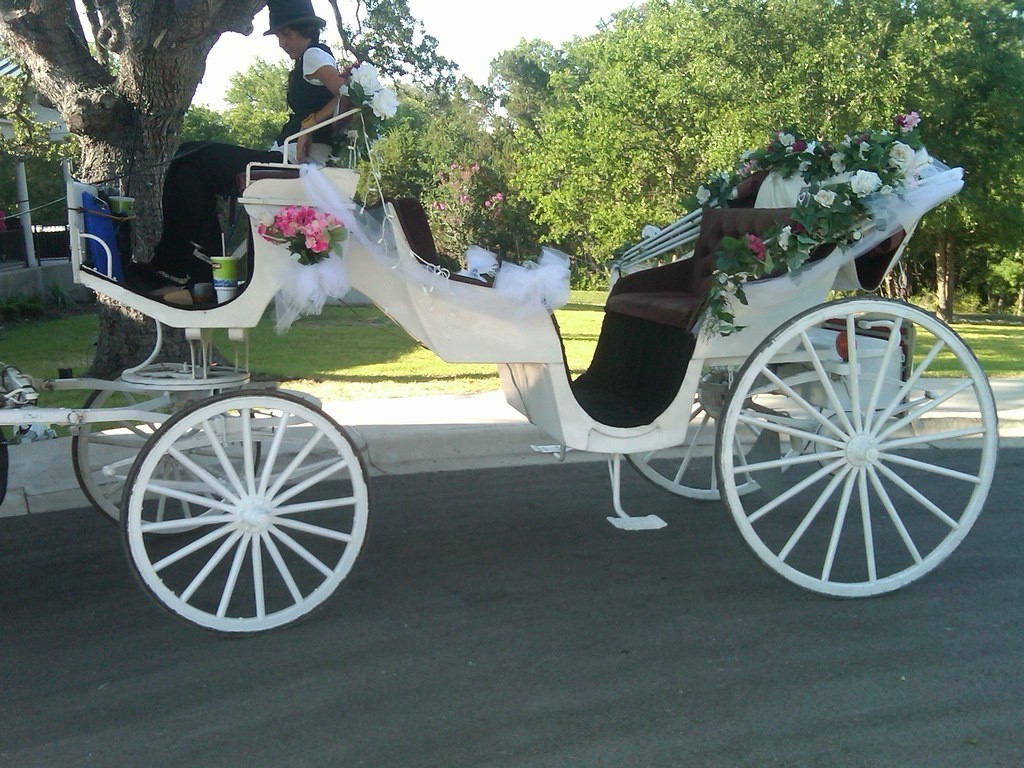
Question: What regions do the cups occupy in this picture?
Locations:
[211,257,238,287]
[108,196,135,216]
[215,287,237,304]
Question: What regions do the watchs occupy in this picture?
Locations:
[301,113,317,129]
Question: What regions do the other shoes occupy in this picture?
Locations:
[154,255,195,284]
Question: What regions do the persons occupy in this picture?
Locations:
[138,0,349,290]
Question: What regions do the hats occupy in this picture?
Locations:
[263,0,326,36]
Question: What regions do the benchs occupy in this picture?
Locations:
[605,183,847,326]
[237,82,364,200]
[378,196,567,363]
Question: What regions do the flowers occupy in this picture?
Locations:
[340,62,400,123]
[256,205,348,274]
[632,110,946,340]
